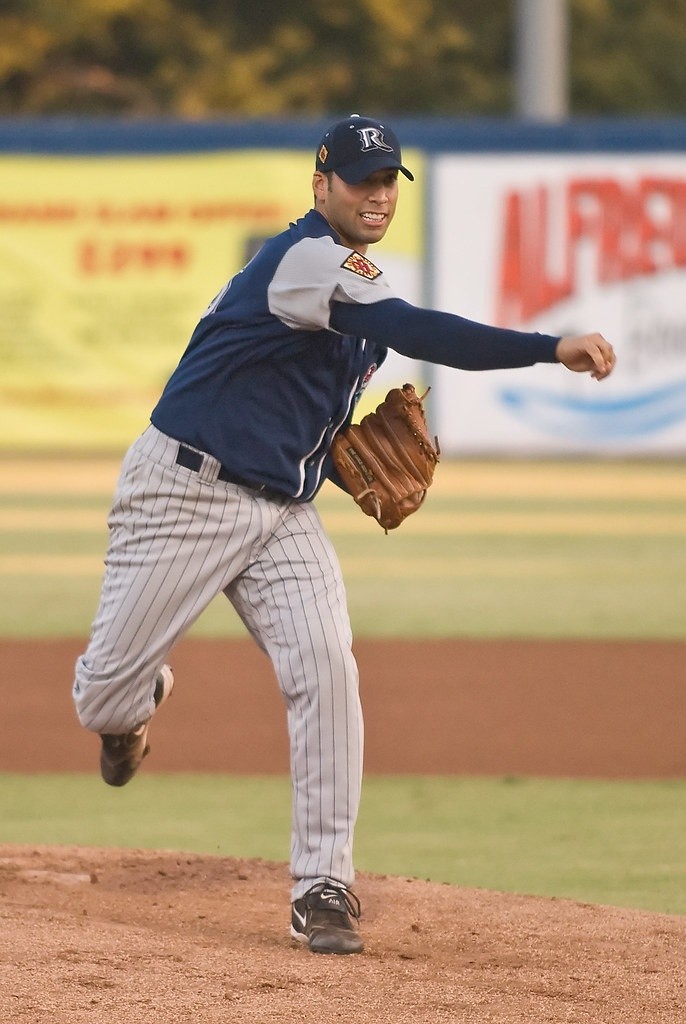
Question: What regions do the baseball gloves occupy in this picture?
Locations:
[331,381,440,532]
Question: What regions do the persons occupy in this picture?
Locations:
[73,114,617,953]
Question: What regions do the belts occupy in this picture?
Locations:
[175,449,289,502]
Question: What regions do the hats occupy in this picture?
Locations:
[314,112,414,186]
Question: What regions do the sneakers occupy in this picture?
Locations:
[100,664,175,786]
[287,883,365,955]
